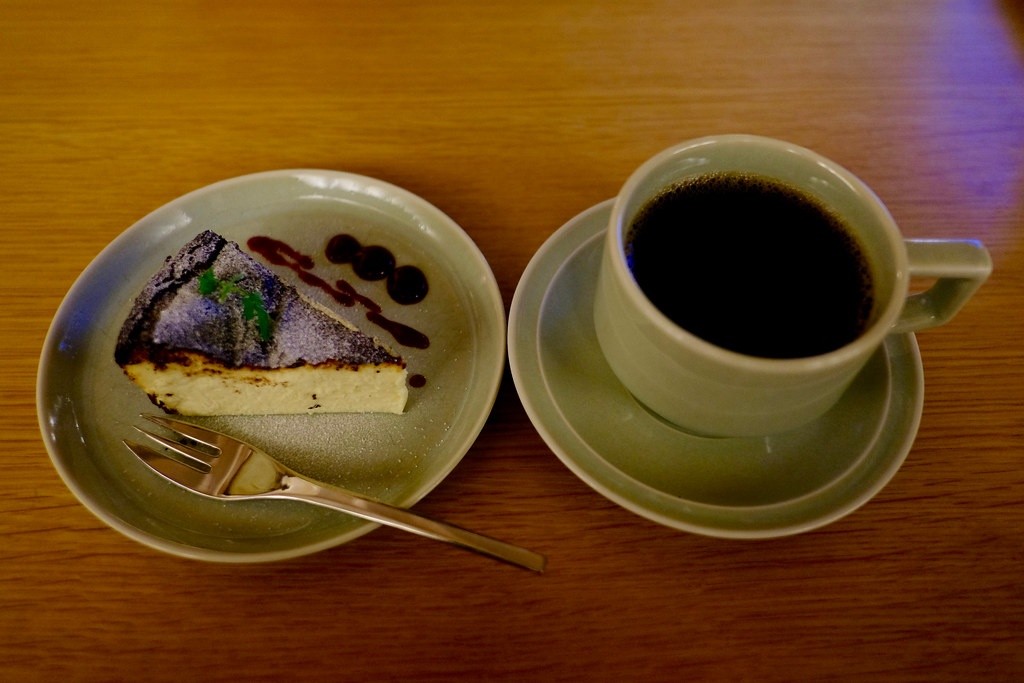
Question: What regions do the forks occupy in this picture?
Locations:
[123,412,549,576]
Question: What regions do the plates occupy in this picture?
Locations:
[508,196,925,540]
[36,167,506,565]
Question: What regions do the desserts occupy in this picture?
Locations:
[112,227,409,417]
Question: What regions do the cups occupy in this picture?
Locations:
[593,133,993,440]
